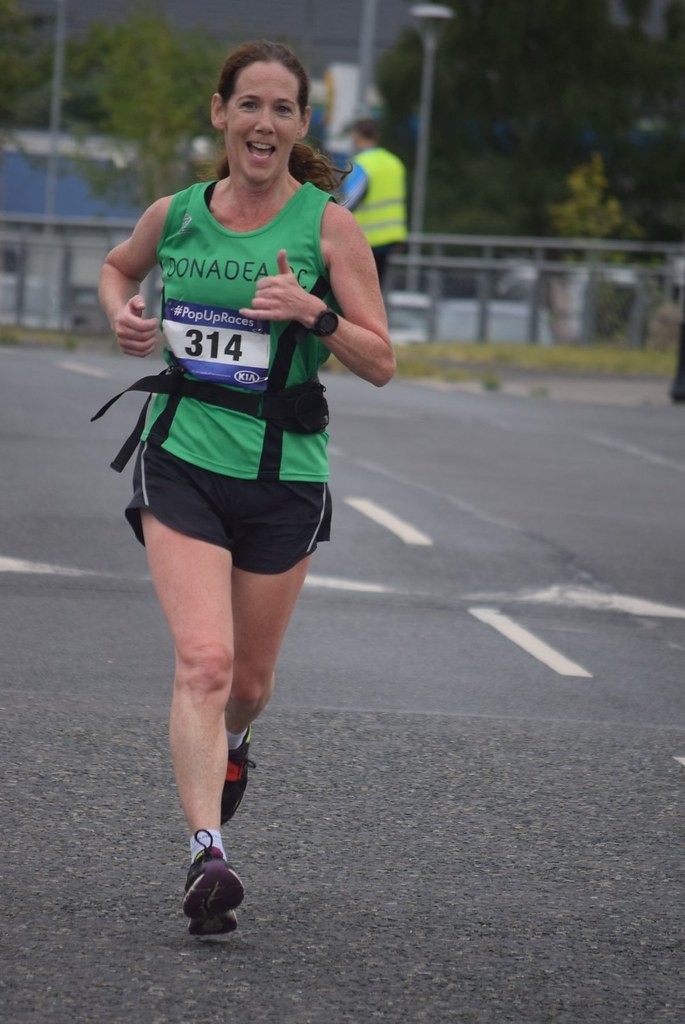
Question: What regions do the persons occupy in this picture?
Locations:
[100,40,408,937]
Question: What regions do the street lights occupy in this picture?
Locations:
[402,4,458,291]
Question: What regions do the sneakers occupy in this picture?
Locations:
[181,829,245,935]
[220,723,256,825]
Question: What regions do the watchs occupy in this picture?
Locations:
[311,303,339,340]
